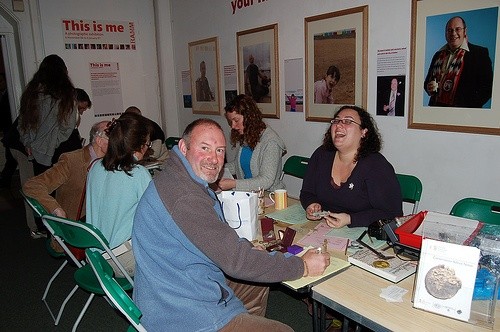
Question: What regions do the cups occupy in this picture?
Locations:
[269,189,288,210]
[249,187,265,215]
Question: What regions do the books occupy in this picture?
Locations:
[348,248,418,283]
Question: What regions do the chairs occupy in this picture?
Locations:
[279,155,500,242]
[19,189,147,332]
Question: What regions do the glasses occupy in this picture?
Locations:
[330,119,363,130]
[446,27,464,34]
[143,140,152,149]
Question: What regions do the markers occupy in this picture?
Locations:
[347,246,363,248]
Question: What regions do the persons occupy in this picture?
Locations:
[22,121,113,253]
[195,61,216,102]
[131,118,330,332]
[381,77,404,116]
[300,96,303,100]
[86,112,153,279]
[314,65,340,104]
[285,93,297,112]
[300,106,403,229]
[424,16,494,109]
[125,105,165,144]
[244,53,268,102]
[215,94,288,192]
[1,54,92,239]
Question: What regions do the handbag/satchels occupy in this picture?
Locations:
[216,190,258,241]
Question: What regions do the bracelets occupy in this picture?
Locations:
[302,258,309,277]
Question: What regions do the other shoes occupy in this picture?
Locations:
[31,228,47,239]
[325,317,355,332]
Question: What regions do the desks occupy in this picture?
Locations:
[257,189,500,332]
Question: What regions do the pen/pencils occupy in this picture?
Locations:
[345,239,349,255]
[368,235,373,243]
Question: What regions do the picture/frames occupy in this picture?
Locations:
[236,23,281,120]
[407,0,500,136]
[303,5,369,123]
[188,36,223,116]
